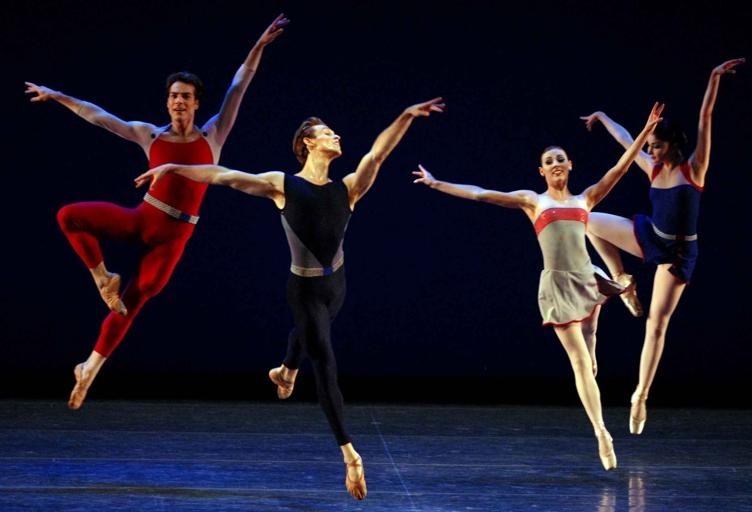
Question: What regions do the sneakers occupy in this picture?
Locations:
[269,366,294,400]
[99,274,129,317]
[614,275,642,316]
[629,388,648,436]
[589,345,598,378]
[69,362,92,411]
[346,455,368,501]
[595,430,618,471]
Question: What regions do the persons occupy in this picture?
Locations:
[134,98,447,501]
[23,13,290,409]
[411,101,667,471]
[579,57,746,434]
